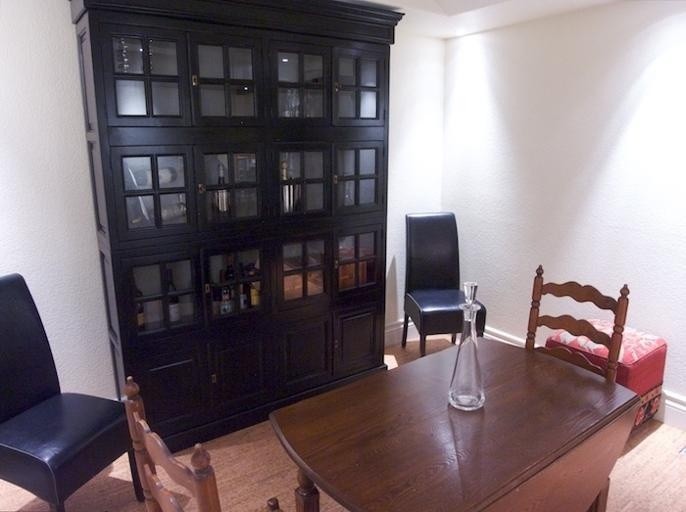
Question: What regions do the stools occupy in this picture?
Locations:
[545,318,664,433]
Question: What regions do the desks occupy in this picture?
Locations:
[263,338,641,511]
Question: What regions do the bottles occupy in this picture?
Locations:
[129,201,188,226]
[447,280,487,411]
[168,267,182,325]
[199,253,262,317]
[125,167,178,189]
[282,160,293,211]
[131,276,147,331]
[215,161,232,221]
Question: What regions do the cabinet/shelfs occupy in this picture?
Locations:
[73,1,406,451]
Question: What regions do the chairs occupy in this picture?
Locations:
[402,212,489,355]
[2,273,145,511]
[525,264,629,381]
[121,373,220,511]
